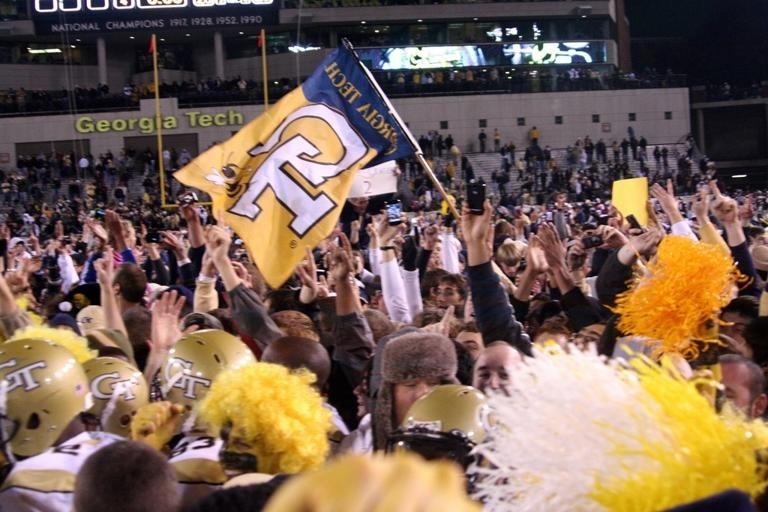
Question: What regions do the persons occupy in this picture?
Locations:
[0,127,768,511]
[376,67,766,106]
[1,73,310,116]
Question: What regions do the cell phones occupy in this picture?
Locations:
[95,209,106,221]
[467,183,485,215]
[626,215,643,234]
[384,200,403,225]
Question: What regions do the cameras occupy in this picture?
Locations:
[145,233,162,243]
[582,234,605,248]
[177,191,199,207]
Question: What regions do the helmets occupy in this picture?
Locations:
[385,384,490,467]
[160,329,257,410]
[83,357,148,439]
[0,338,96,458]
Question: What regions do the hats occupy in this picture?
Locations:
[368,326,464,452]
[77,305,108,337]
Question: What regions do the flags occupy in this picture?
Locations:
[169,36,459,295]
